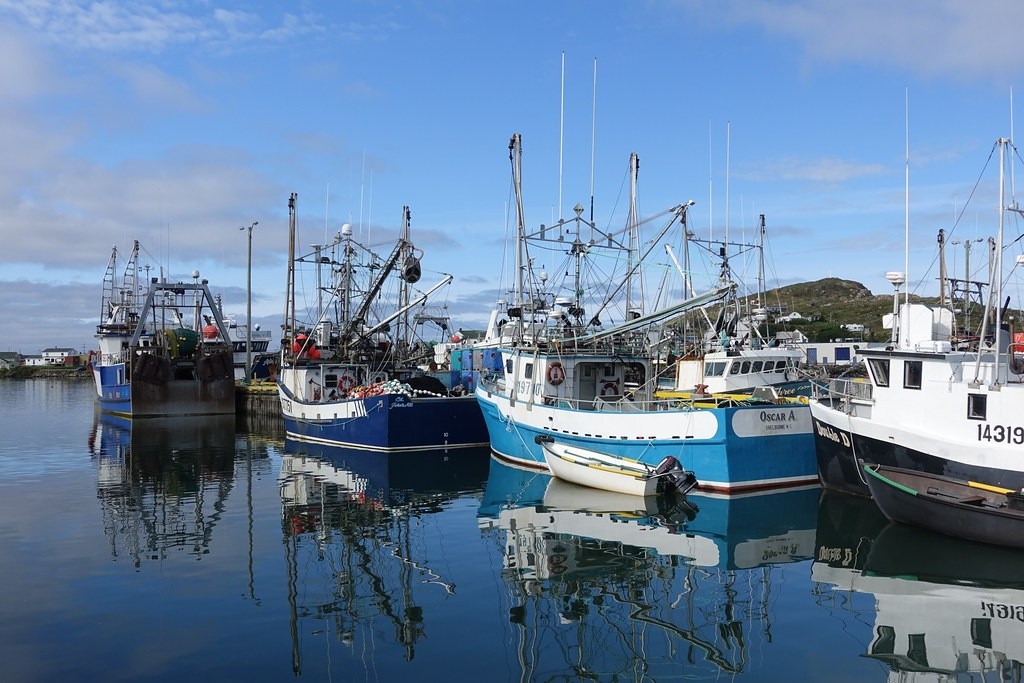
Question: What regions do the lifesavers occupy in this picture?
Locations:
[339,376,353,392]
[546,362,566,385]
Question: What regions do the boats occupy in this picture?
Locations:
[656,81,1024,498]
[533,432,700,494]
[855,457,1024,550]
[807,485,1024,683]
[275,162,494,450]
[187,268,273,380]
[863,522,1024,596]
[542,472,698,525]
[469,48,819,494]
[86,237,239,416]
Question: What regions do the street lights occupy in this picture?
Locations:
[239,220,260,386]
